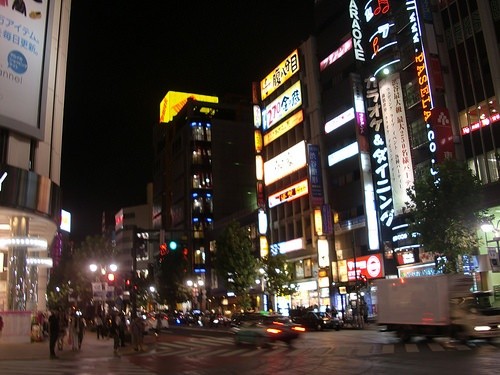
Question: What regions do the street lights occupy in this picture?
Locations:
[480,215,500,261]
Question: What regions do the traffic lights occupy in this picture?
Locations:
[160,240,187,261]
[106,273,116,282]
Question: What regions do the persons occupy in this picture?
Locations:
[155,313,161,336]
[0,315,4,331]
[332,307,338,318]
[95,311,128,352]
[129,315,146,351]
[38,310,49,336]
[48,307,87,360]
[344,302,369,328]
[326,305,331,313]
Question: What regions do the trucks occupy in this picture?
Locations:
[377,273,500,343]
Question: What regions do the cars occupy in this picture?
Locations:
[124,311,169,337]
[231,311,305,343]
[293,312,343,331]
